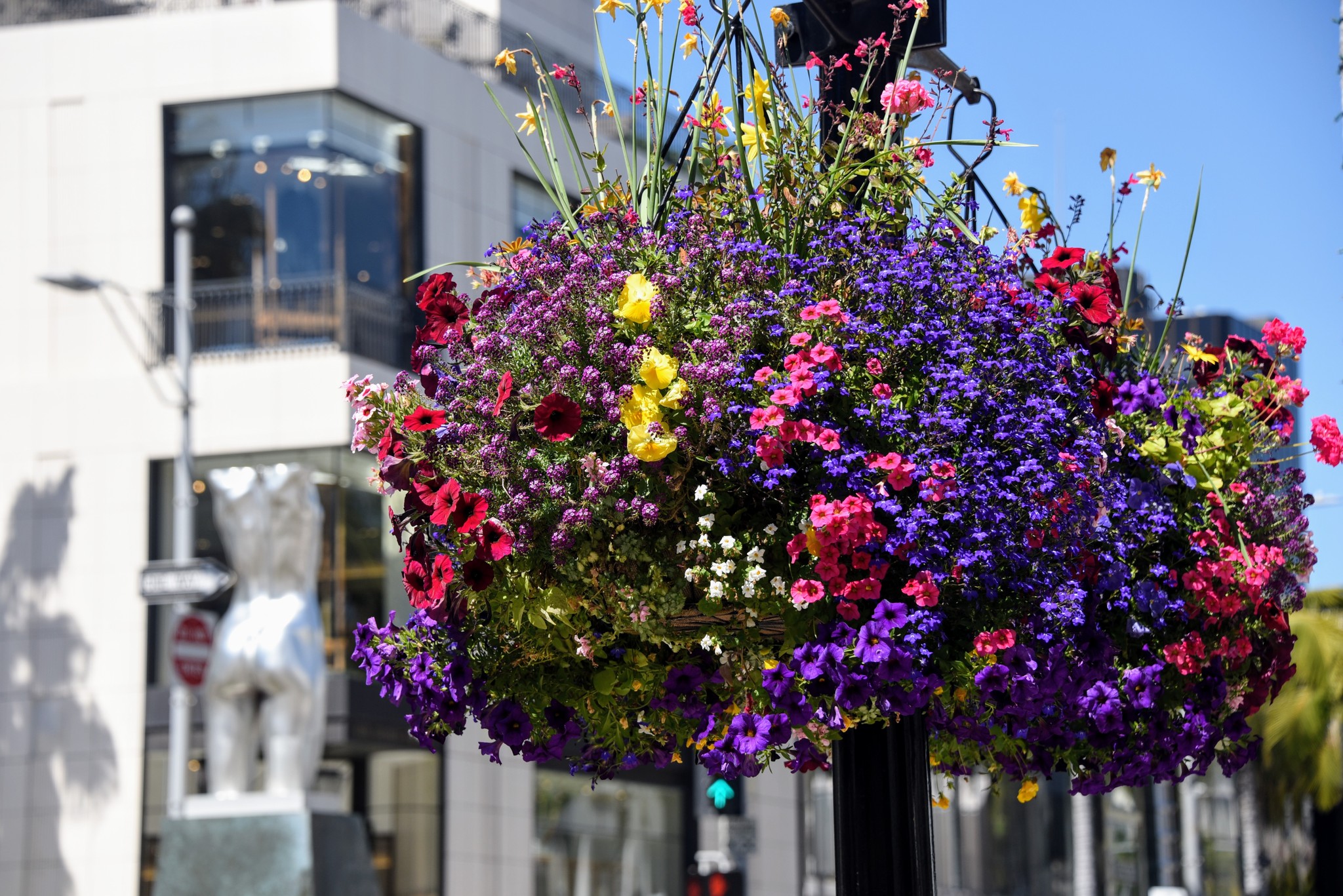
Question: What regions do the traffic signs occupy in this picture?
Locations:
[139,559,239,605]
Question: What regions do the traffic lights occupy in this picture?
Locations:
[686,868,745,896]
[707,771,744,816]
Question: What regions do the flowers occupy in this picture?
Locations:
[355,0,1343,791]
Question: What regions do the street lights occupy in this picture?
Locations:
[33,203,195,819]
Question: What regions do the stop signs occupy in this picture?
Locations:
[171,610,223,687]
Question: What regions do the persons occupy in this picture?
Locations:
[203,467,326,796]
